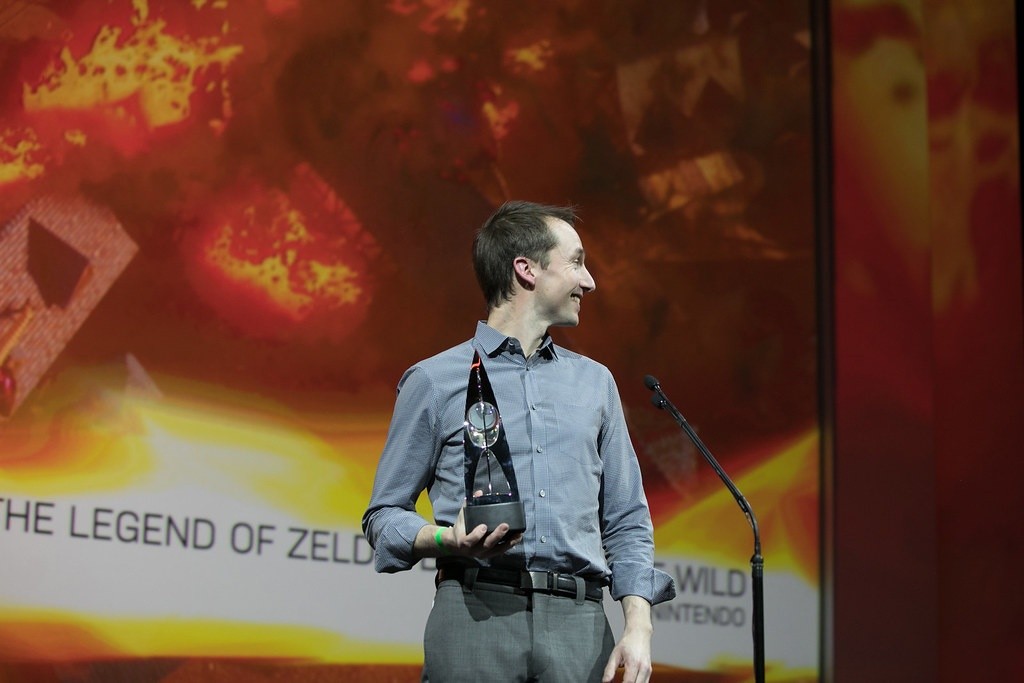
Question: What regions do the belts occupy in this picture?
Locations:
[437,567,603,601]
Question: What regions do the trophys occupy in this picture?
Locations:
[462,347,527,534]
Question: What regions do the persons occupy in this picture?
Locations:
[362,202,676,683]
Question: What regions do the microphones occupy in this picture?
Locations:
[644,374,760,554]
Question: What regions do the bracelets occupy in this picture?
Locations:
[435,527,451,556]
